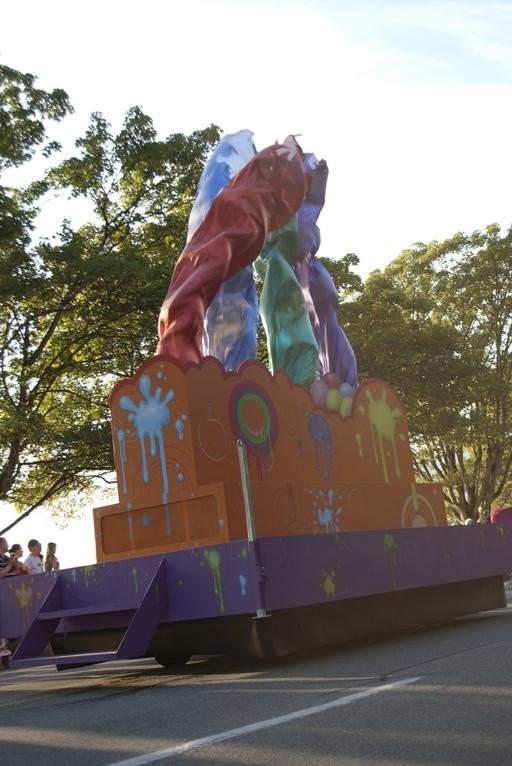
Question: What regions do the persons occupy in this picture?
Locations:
[446,503,485,527]
[0,537,59,579]
